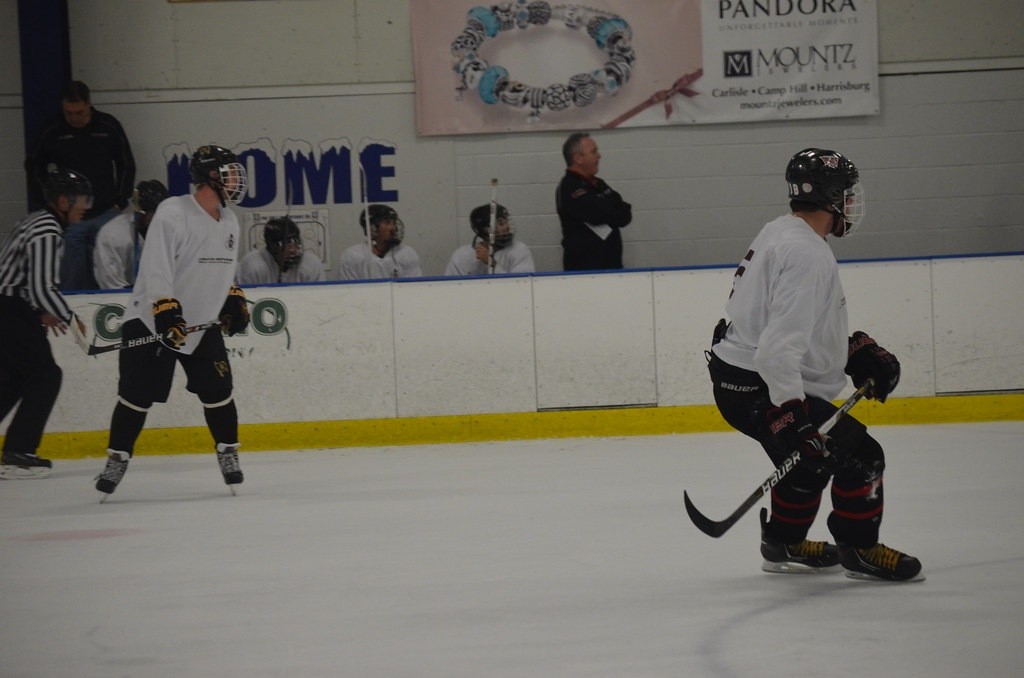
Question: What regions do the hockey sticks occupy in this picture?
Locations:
[69,313,251,356]
[682,378,876,539]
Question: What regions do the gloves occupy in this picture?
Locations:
[844,330,900,403]
[767,398,837,474]
[154,297,191,351]
[218,284,252,338]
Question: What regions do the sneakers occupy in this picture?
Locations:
[92,448,131,503]
[0,442,51,482]
[838,535,924,585]
[213,442,244,498]
[757,507,848,576]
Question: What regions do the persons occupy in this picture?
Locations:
[234,216,326,286]
[704,146,923,583]
[93,179,169,290]
[96,145,249,491]
[31,79,137,290]
[0,168,91,469]
[443,203,535,276]
[555,133,632,271]
[338,204,422,280]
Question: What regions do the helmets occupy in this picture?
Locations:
[360,203,405,247]
[136,179,167,209]
[190,145,247,207]
[42,169,92,207]
[264,214,303,264]
[470,205,514,248]
[783,145,866,239]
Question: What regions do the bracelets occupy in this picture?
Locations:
[450,0,637,125]
